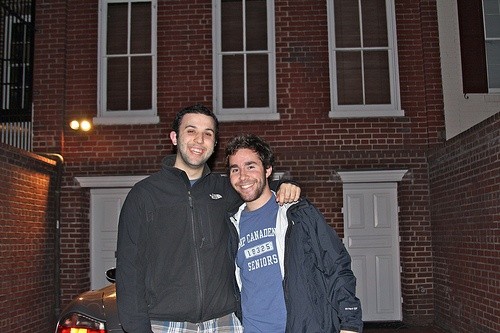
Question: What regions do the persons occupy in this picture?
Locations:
[115,106,302,333]
[224,135,363,333]
